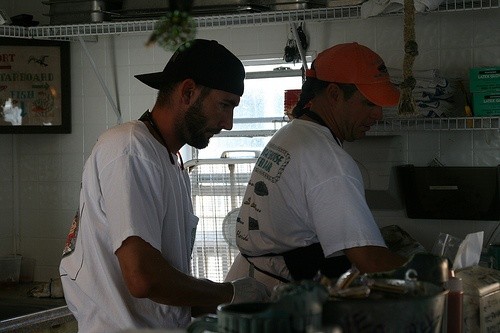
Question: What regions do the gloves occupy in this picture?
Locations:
[225,277,267,305]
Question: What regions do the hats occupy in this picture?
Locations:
[306,42,402,108]
[134,39,245,96]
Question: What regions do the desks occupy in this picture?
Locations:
[0,285,75,333]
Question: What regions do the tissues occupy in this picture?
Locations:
[452,231,500,333]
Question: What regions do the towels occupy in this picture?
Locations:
[361,0,441,20]
[382,68,457,127]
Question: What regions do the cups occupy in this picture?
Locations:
[273,281,322,333]
[217,299,276,332]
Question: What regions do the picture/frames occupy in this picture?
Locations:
[0,37,72,134]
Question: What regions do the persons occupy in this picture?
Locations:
[59,38,273,333]
[224,42,408,294]
